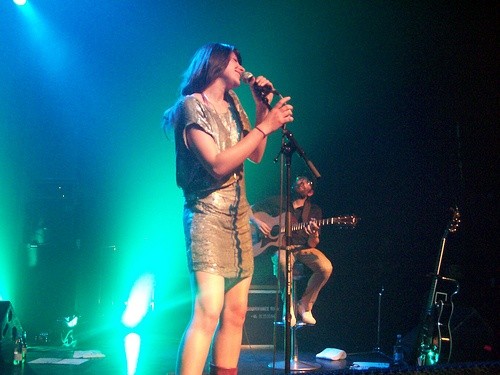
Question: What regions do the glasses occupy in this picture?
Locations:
[296,176,313,186]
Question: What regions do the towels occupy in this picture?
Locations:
[315,347,347,361]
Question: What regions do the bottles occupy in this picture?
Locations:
[393,334,404,366]
[13,338,22,366]
[19,335,28,362]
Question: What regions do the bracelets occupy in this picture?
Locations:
[255,126,266,137]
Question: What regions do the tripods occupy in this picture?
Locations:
[345,288,393,363]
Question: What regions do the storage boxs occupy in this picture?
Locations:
[241,290,283,349]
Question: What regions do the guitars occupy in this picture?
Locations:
[250,210,358,259]
[399,206,461,368]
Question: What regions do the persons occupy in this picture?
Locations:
[248,172,332,324]
[163,42,293,374]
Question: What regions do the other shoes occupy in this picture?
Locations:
[282,305,296,327]
[296,303,317,324]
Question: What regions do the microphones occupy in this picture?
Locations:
[243,72,278,94]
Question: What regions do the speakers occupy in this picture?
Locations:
[0,301,23,367]
